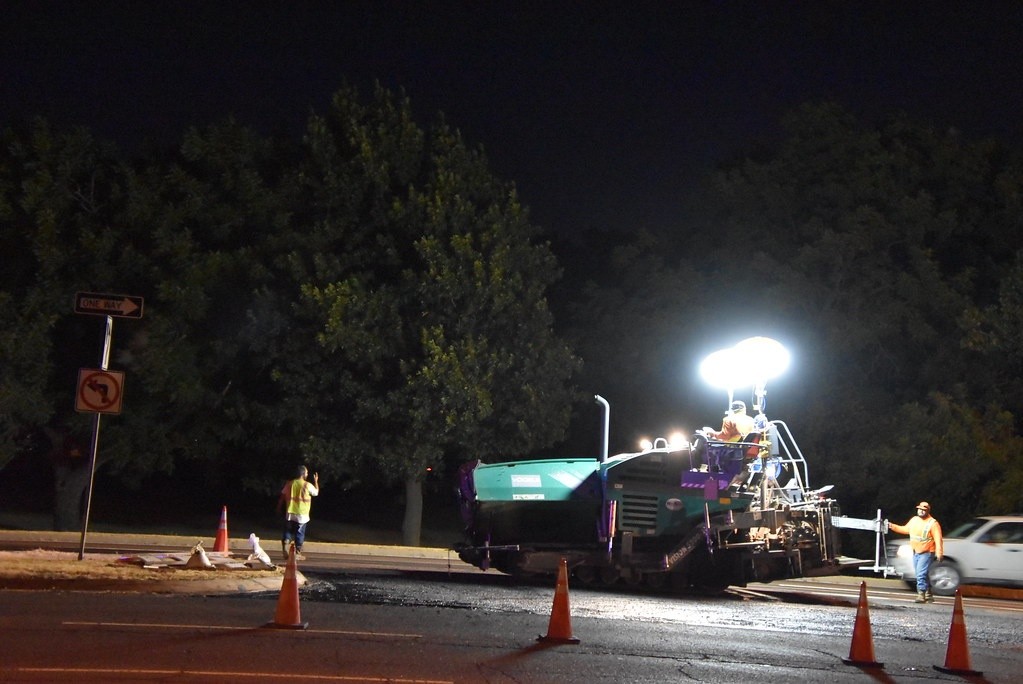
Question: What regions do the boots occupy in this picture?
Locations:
[915,590,925,603]
[296,549,306,561]
[924,589,933,602]
[282,539,290,559]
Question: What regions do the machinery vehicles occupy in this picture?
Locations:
[451,338,838,596]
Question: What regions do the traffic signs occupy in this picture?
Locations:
[74,291,143,318]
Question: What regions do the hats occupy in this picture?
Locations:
[731,400,745,411]
[916,502,930,511]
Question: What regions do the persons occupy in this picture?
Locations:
[276,466,319,560]
[702,400,754,444]
[887,501,943,604]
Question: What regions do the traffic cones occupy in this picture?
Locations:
[212,506,229,557]
[267,543,309,630]
[539,557,581,644]
[933,588,983,677]
[841,581,885,668]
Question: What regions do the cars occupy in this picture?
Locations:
[880,516,1023,595]
